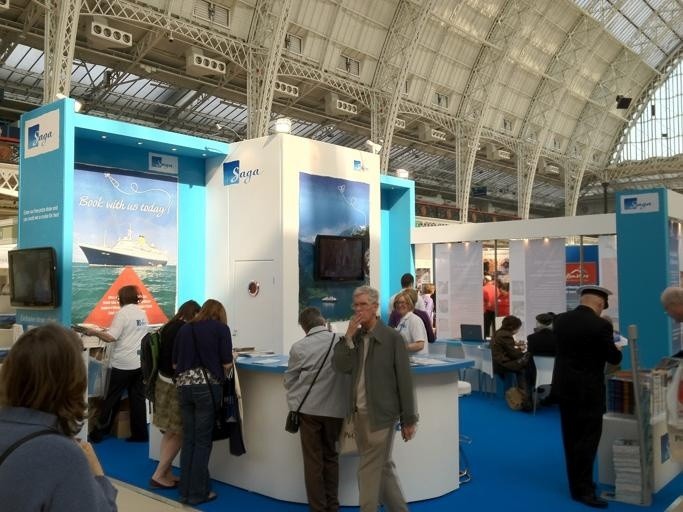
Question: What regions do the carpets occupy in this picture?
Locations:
[89,391,683,512]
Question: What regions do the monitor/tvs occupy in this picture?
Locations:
[7,247,58,311]
[313,234,366,283]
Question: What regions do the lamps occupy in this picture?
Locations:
[487,146,510,160]
[273,81,299,98]
[539,160,558,174]
[419,125,445,141]
[169,32,174,42]
[185,45,226,76]
[324,93,357,116]
[616,95,632,109]
[216,124,243,140]
[394,117,405,130]
[86,15,132,49]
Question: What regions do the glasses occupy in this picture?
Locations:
[663,310,667,315]
[351,299,376,311]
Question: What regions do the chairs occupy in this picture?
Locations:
[429,341,554,416]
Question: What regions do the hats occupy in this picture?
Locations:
[536,312,555,325]
[575,285,612,309]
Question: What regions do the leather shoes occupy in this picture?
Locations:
[151,475,217,505]
[572,495,607,507]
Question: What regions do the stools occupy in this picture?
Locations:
[458,382,472,484]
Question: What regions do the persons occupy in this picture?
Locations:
[282,310,353,511]
[553,285,623,507]
[659,286,682,323]
[0,321,120,512]
[333,283,419,511]
[70,284,148,443]
[140,299,201,487]
[389,260,555,417]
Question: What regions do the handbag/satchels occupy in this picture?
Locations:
[213,409,230,441]
[285,412,300,433]
[505,387,531,410]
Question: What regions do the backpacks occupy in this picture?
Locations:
[141,332,160,401]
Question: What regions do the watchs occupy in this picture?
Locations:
[170,300,237,506]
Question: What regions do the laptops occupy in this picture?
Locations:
[460,324,484,343]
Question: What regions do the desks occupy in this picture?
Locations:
[598,412,682,494]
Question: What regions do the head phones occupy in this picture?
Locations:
[130,284,143,304]
